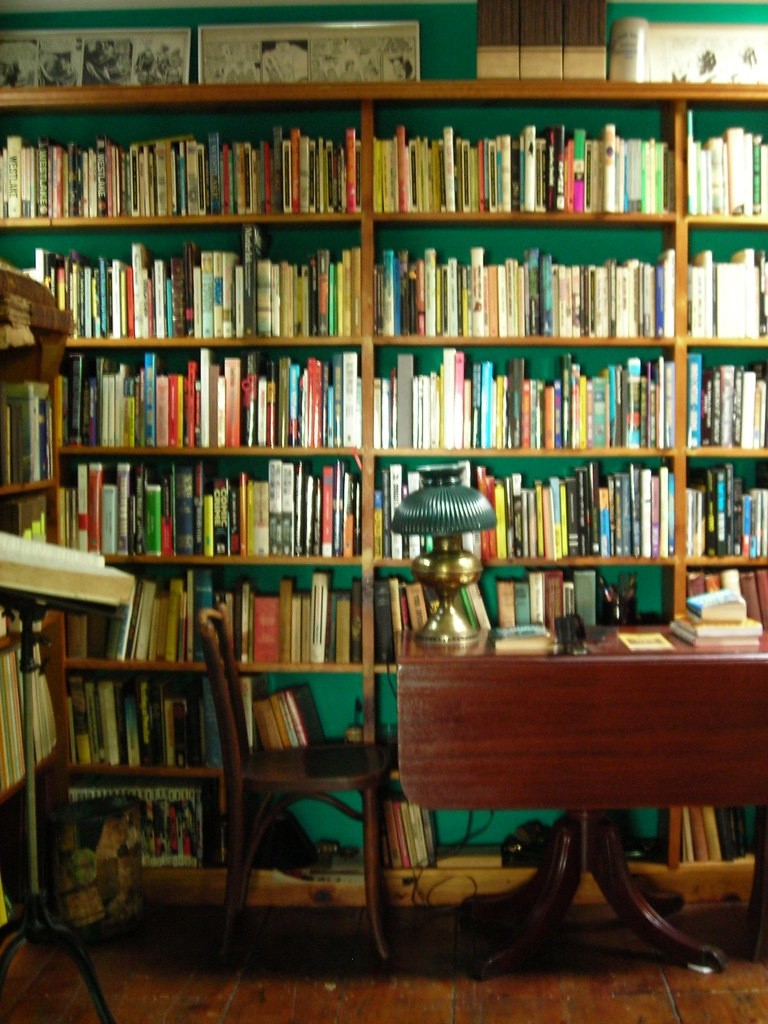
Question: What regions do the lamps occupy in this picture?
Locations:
[390,466,499,640]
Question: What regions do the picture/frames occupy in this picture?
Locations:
[0,28,192,87]
[197,21,421,84]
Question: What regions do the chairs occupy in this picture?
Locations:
[198,607,400,966]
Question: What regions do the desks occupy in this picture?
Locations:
[398,628,767,979]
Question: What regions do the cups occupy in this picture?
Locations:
[604,598,637,627]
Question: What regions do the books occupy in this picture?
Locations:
[0,123,768,868]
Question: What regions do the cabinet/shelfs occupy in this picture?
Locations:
[0,88,768,980]
